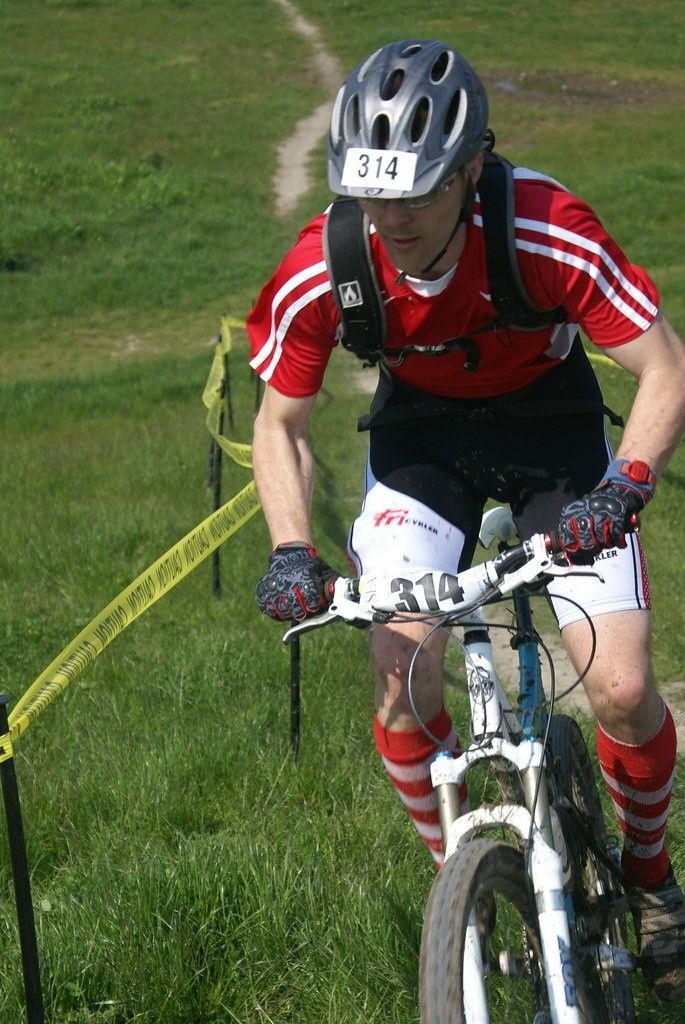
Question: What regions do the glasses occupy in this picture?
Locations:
[358,167,459,209]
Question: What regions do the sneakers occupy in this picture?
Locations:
[629,865,684,1004]
[473,886,496,939]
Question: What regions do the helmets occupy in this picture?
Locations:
[326,40,489,198]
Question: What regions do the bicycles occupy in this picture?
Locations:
[278,512,639,1023]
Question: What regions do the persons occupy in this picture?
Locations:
[246,41,684,1008]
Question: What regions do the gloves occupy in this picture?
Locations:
[257,541,339,618]
[559,458,656,552]
